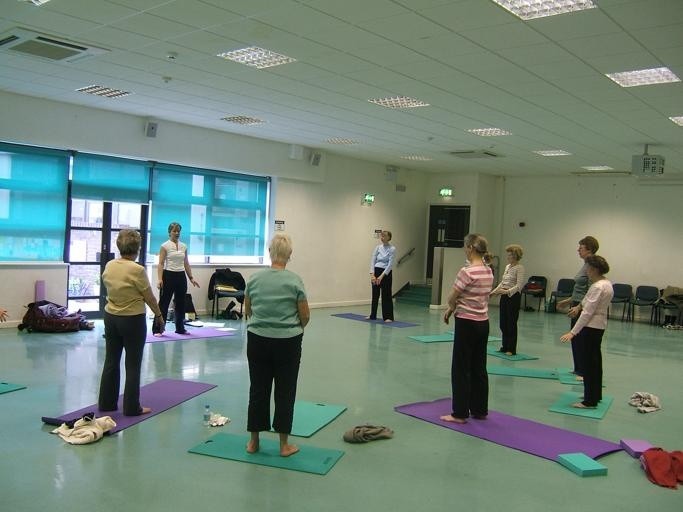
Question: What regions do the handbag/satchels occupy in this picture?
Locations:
[189,276,193,281]
[153,312,163,318]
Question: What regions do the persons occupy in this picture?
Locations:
[241,234,312,460]
[152,221,200,339]
[488,244,526,356]
[364,229,397,324]
[437,232,495,424]
[95,225,166,418]
[558,254,615,410]
[555,235,599,381]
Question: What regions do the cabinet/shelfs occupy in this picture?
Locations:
[203,405,212,430]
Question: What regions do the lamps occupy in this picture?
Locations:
[653,285,683,326]
[212,268,244,320]
[629,285,659,325]
[549,279,575,312]
[521,275,547,313]
[608,283,633,322]
